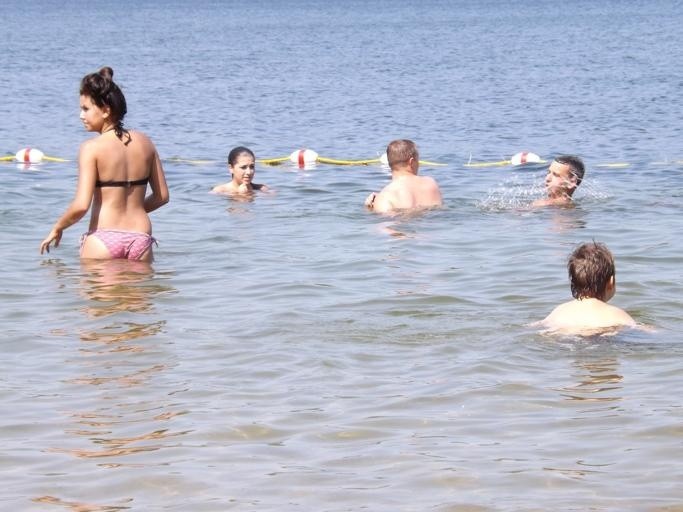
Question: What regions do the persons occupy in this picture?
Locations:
[207,147,270,196]
[40,66,169,261]
[522,243,664,334]
[364,140,443,216]
[532,155,585,206]
